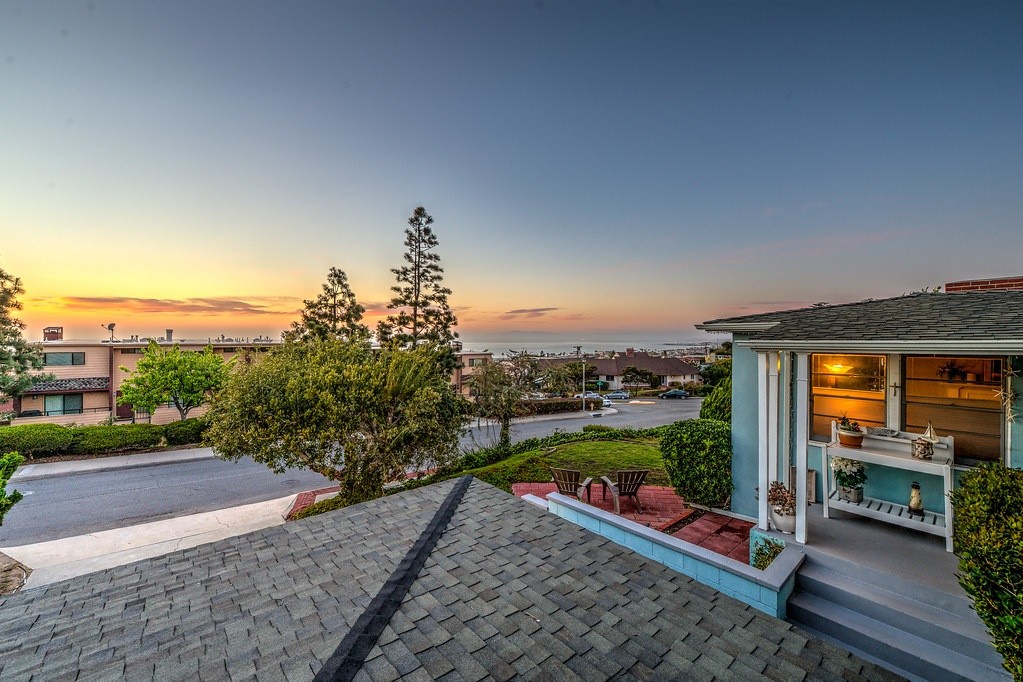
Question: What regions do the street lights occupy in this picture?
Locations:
[580,355,586,411]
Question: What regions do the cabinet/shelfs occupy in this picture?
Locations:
[822,420,955,553]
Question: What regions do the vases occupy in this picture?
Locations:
[839,432,865,446]
[839,485,863,503]
[770,503,796,535]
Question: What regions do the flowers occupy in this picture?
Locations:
[829,455,868,489]
[755,481,811,516]
[838,410,861,432]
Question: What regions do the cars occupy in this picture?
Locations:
[595,395,613,407]
[573,391,601,398]
[603,389,634,400]
[657,389,691,399]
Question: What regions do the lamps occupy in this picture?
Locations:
[822,364,854,388]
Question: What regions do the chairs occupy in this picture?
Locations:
[549,466,593,504]
[600,470,651,516]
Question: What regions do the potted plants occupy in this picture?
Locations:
[936,360,968,383]
